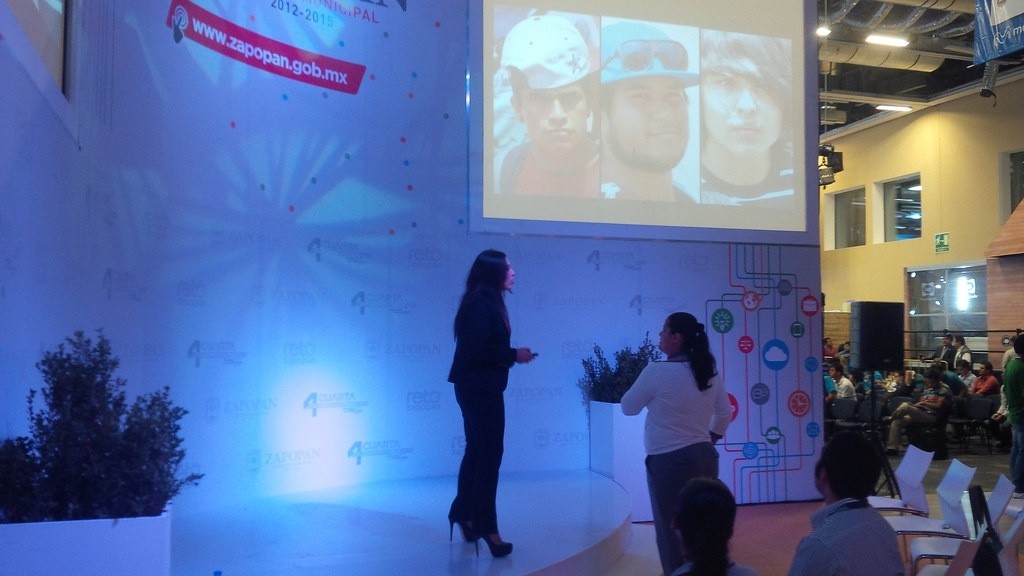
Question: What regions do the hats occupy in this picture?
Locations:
[601,21,699,87]
[500,15,589,100]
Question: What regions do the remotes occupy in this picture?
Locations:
[533,352,538,355]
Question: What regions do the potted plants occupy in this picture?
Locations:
[581,333,653,523]
[0,325,204,576]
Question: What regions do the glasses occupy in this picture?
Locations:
[602,39,689,70]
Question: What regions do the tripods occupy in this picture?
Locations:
[866,370,901,500]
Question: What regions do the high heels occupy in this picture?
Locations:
[473,531,514,557]
[448,495,482,543]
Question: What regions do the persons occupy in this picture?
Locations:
[787,432,906,576]
[700,31,801,216]
[822,333,1024,498]
[668,477,759,576]
[602,37,703,205]
[498,13,604,204]
[621,311,738,576]
[446,249,539,557]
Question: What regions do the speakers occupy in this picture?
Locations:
[849,302,904,372]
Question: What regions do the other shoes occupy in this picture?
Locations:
[882,415,891,425]
[1013,492,1024,499]
[884,449,898,457]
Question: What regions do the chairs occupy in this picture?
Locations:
[825,393,1024,576]
